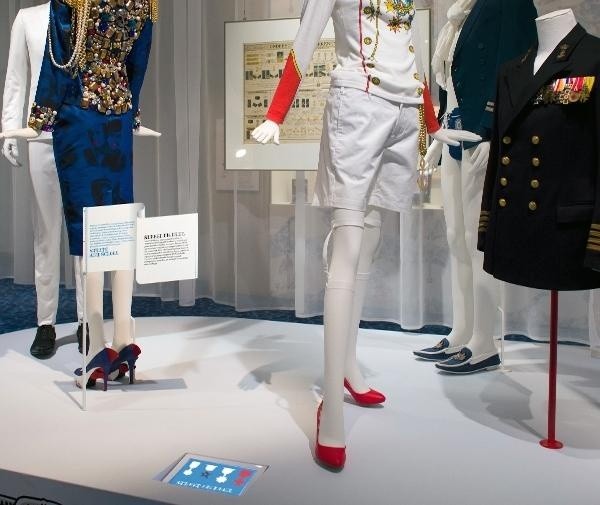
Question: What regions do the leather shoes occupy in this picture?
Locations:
[77,323,90,356]
[30,325,56,359]
[413,338,500,374]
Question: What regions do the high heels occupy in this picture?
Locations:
[74,344,141,391]
[315,400,347,470]
[344,378,386,406]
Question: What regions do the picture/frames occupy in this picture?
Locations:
[219,7,433,174]
